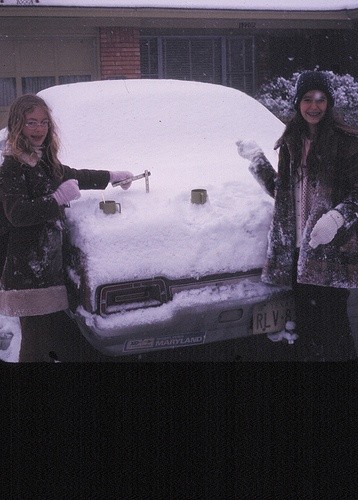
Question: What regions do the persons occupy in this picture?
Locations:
[235,71,358,373]
[0,94,135,377]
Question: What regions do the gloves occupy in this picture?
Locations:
[237,138,263,160]
[307,211,339,248]
[110,172,133,190]
[55,179,80,206]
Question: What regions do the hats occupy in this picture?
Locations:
[292,72,334,108]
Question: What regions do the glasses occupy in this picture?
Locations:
[23,120,50,129]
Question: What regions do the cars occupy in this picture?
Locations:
[0,79,288,357]
